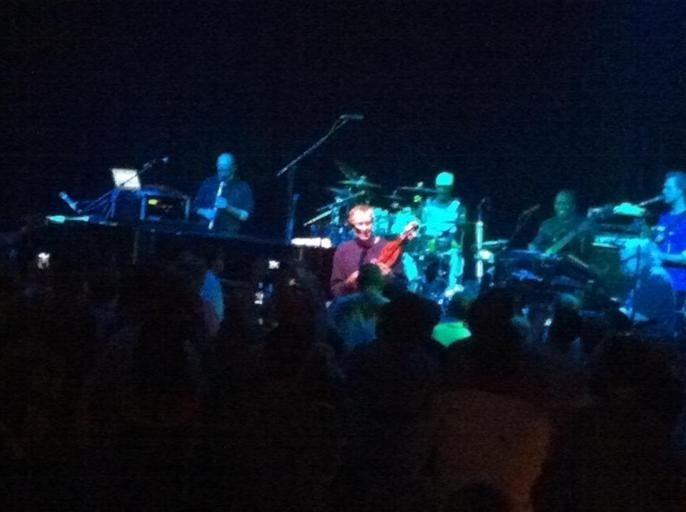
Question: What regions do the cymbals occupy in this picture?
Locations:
[331,188,356,198]
[339,180,379,187]
[384,194,405,200]
[401,187,440,193]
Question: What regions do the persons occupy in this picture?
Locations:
[192,152,258,234]
[330,202,417,293]
[649,172,686,301]
[1,269,686,511]
[622,240,674,332]
[421,172,466,280]
[529,189,584,253]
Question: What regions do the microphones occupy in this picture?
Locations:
[339,114,364,121]
[640,193,664,206]
[523,204,541,215]
[143,157,169,168]
